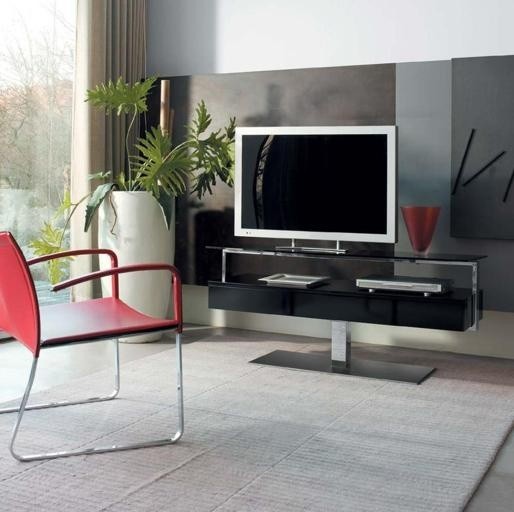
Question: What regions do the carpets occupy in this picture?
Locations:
[0,328,513,512]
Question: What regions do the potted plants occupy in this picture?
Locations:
[23,75,239,345]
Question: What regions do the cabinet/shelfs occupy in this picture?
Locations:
[202,239,485,385]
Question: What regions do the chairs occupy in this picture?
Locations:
[0,230,187,463]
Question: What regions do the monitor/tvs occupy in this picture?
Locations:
[233,124,399,255]
